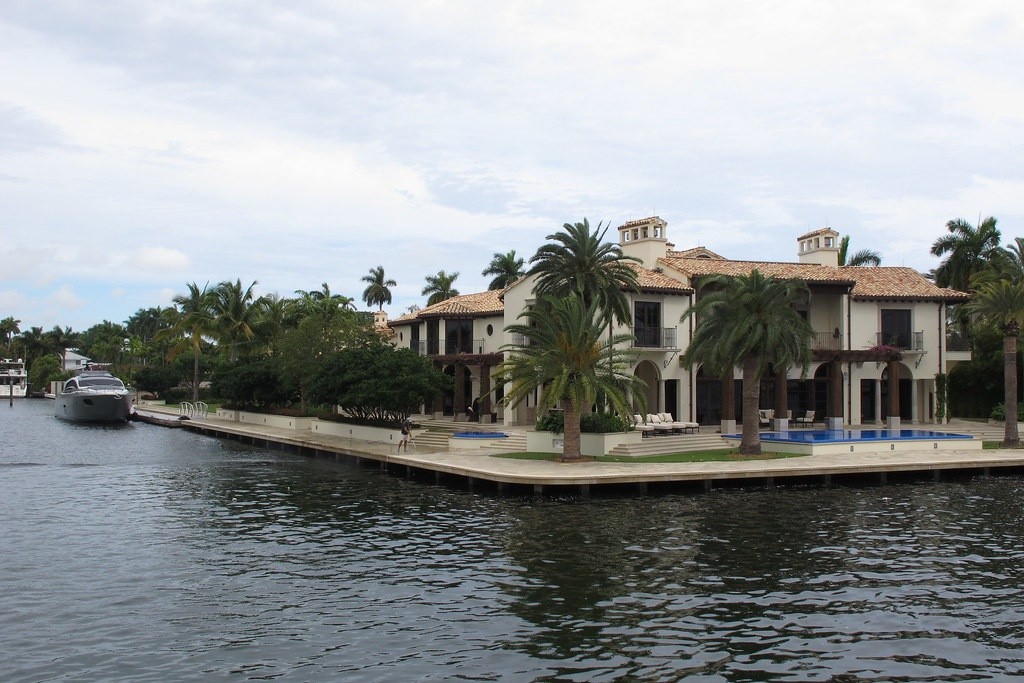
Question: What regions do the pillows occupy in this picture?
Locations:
[634,413,674,423]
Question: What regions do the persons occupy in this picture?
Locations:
[396,416,412,453]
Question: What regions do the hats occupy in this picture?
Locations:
[407,418,412,422]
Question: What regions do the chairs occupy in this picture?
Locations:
[628,413,700,437]
[757,409,792,423]
[795,410,816,428]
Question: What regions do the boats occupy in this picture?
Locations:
[54,363,130,423]
[0,358,28,399]
